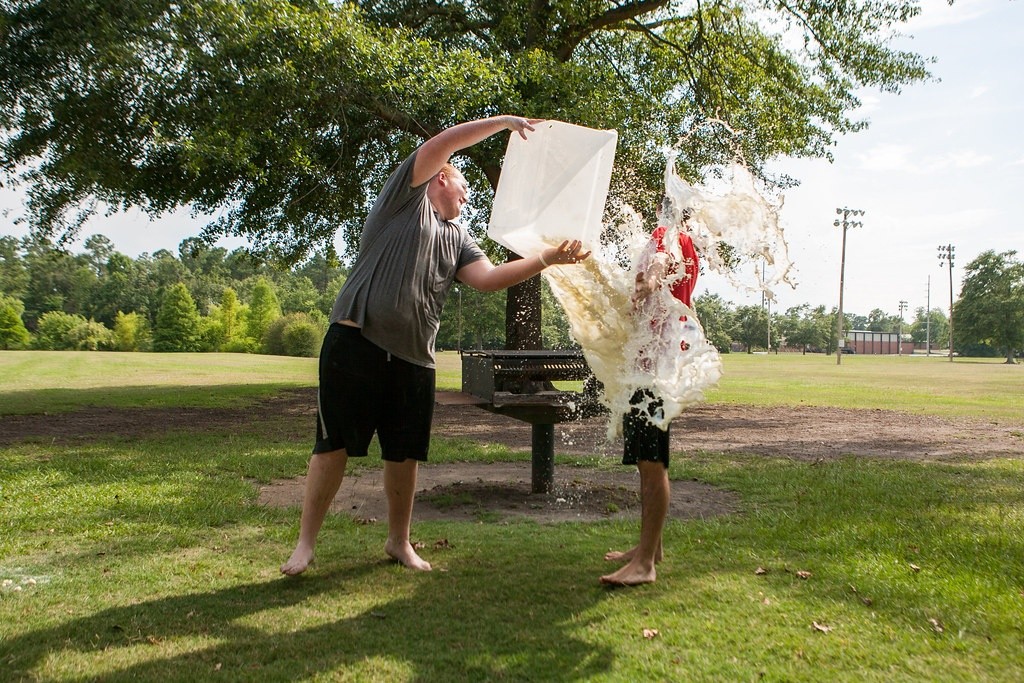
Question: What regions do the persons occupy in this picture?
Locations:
[280,115,592,576]
[599,186,699,584]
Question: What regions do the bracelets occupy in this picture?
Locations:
[538,252,550,269]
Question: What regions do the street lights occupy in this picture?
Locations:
[453,288,460,355]
[833,206,866,365]
[898,300,909,356]
[937,243,955,361]
